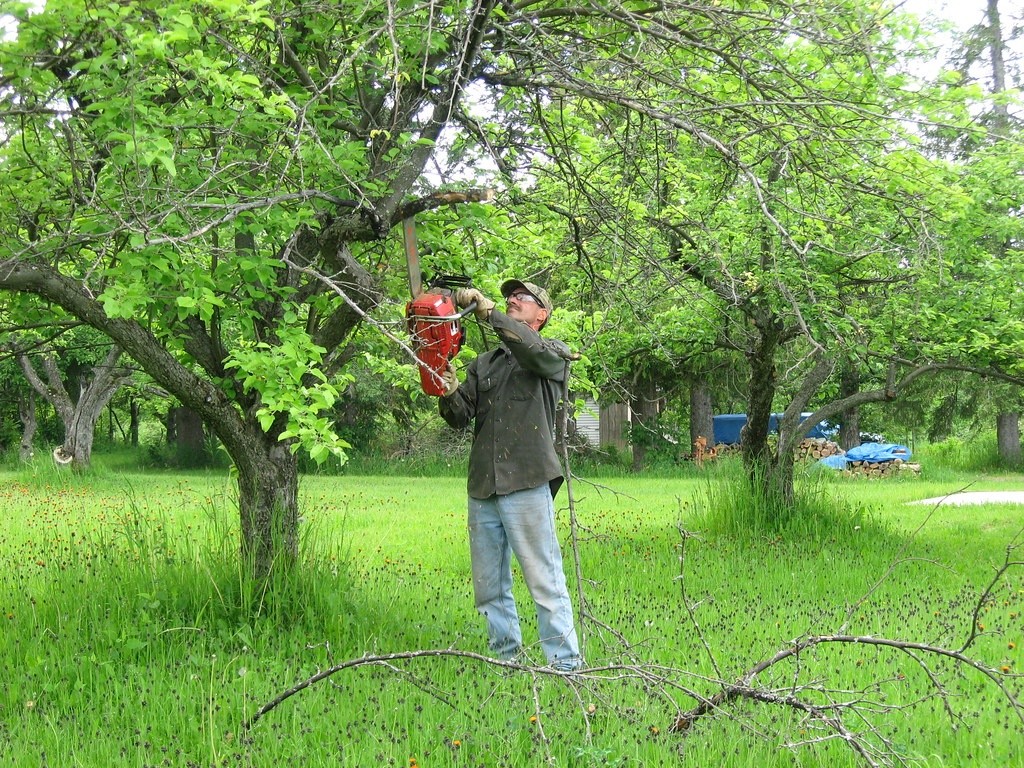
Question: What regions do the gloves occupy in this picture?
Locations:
[455,288,495,320]
[439,363,459,396]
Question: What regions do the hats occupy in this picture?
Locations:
[501,279,553,324]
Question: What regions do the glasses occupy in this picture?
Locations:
[505,292,536,303]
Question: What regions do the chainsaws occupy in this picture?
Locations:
[399,197,481,399]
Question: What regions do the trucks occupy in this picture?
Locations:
[713,412,886,450]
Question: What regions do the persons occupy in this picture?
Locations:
[438,279,582,672]
